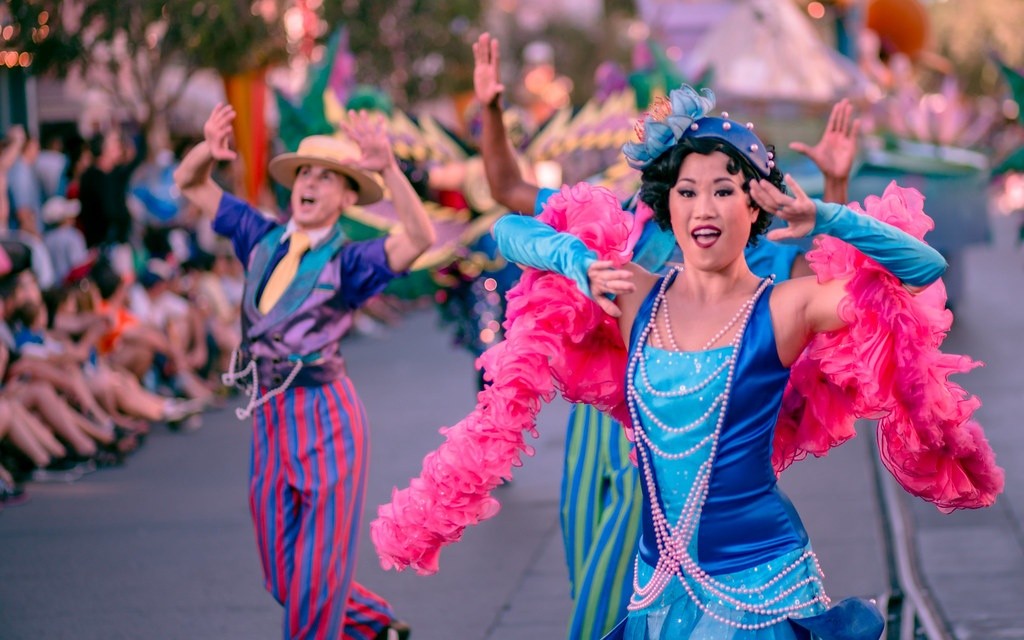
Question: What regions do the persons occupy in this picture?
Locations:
[469,27,865,613]
[173,103,441,639]
[489,111,948,640]
[0,0,1023,505]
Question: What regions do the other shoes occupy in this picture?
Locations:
[373,621,411,640]
[1,393,203,507]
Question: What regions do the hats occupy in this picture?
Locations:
[269,135,382,205]
